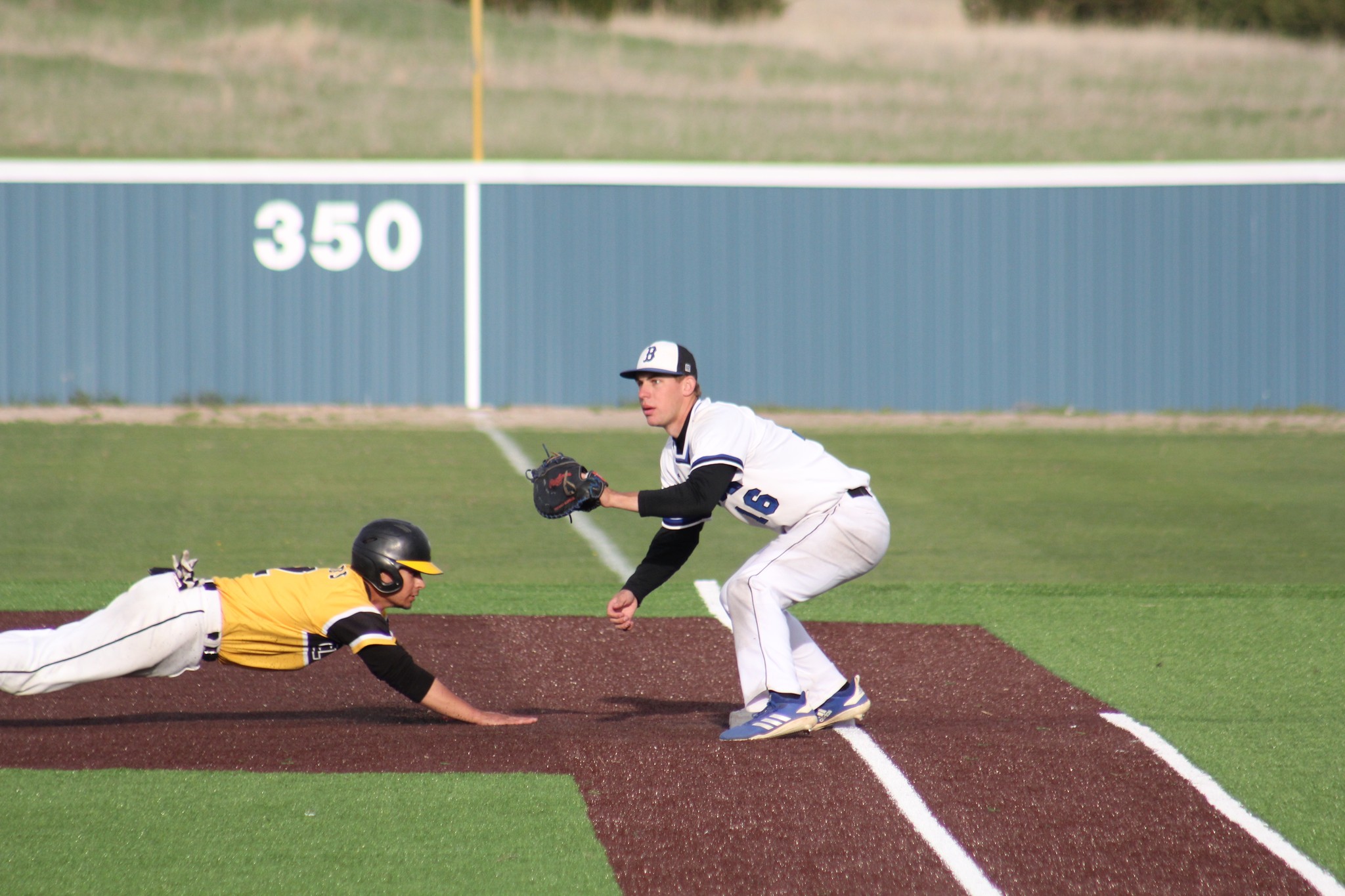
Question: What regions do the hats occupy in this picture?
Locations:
[619,340,698,380]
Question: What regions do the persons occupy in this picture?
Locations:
[0,518,538,726]
[533,340,892,742]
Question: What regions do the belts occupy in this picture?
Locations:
[781,487,872,534]
[203,581,220,664]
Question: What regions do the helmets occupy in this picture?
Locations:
[350,517,444,593]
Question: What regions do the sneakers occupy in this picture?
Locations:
[718,692,818,741]
[808,674,871,732]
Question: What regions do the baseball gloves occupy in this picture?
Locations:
[524,443,609,523]
